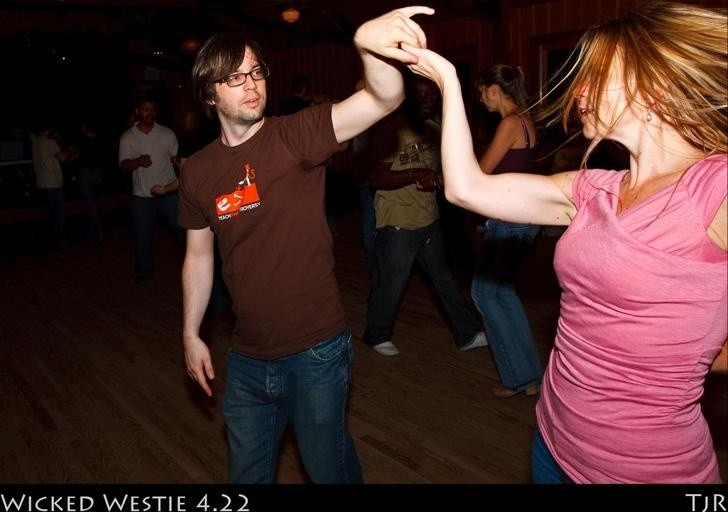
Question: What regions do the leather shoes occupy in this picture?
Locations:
[459,332,488,351]
[373,341,399,356]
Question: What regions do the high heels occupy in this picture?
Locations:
[494,380,541,397]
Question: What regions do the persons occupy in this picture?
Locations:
[33,129,69,220]
[178,6,435,484]
[400,0,728,484]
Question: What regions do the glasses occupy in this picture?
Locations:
[213,66,270,87]
